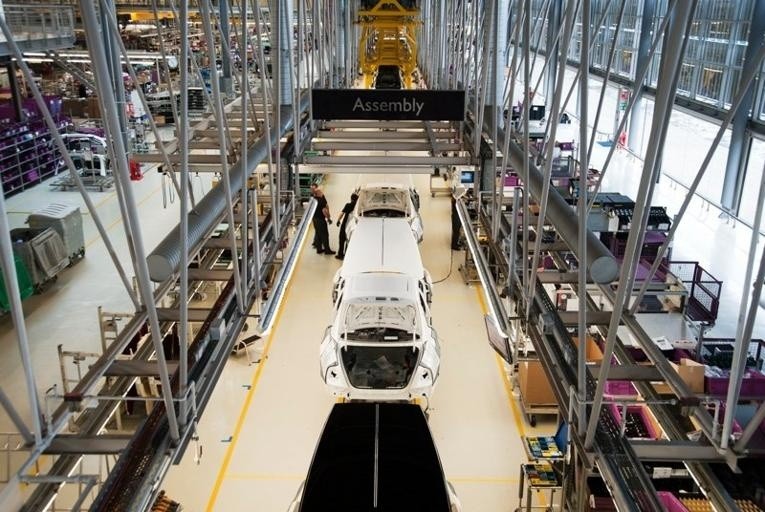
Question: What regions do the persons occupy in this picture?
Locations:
[312,189,336,255]
[335,193,359,261]
[310,183,329,249]
[451,197,462,250]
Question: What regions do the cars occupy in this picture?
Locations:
[287,401,460,512]
[317,184,442,413]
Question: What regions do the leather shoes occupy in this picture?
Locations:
[336,256,343,260]
[452,244,461,250]
[318,250,324,253]
[325,249,336,254]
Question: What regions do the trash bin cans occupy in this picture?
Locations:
[0,250,34,316]
[24,202,86,269]
[9,227,71,295]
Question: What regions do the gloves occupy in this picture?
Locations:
[327,219,332,224]
[336,221,340,227]
